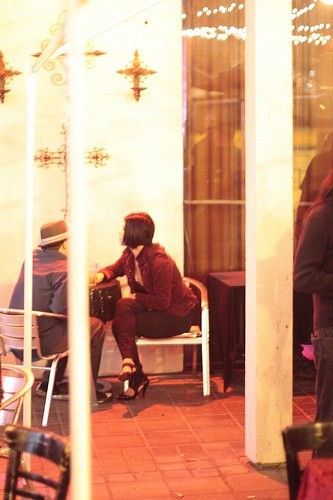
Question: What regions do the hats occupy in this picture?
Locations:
[37,219,72,248]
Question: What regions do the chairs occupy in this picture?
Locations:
[120,277,213,398]
[0,305,100,428]
[2,426,77,499]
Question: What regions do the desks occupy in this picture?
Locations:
[206,270,316,393]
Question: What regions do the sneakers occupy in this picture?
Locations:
[34,381,60,398]
[93,390,107,402]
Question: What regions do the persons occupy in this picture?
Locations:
[93,213,198,400]
[294,166,333,458]
[7,220,113,404]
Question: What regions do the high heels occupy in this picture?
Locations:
[119,358,143,383]
[116,379,149,400]
